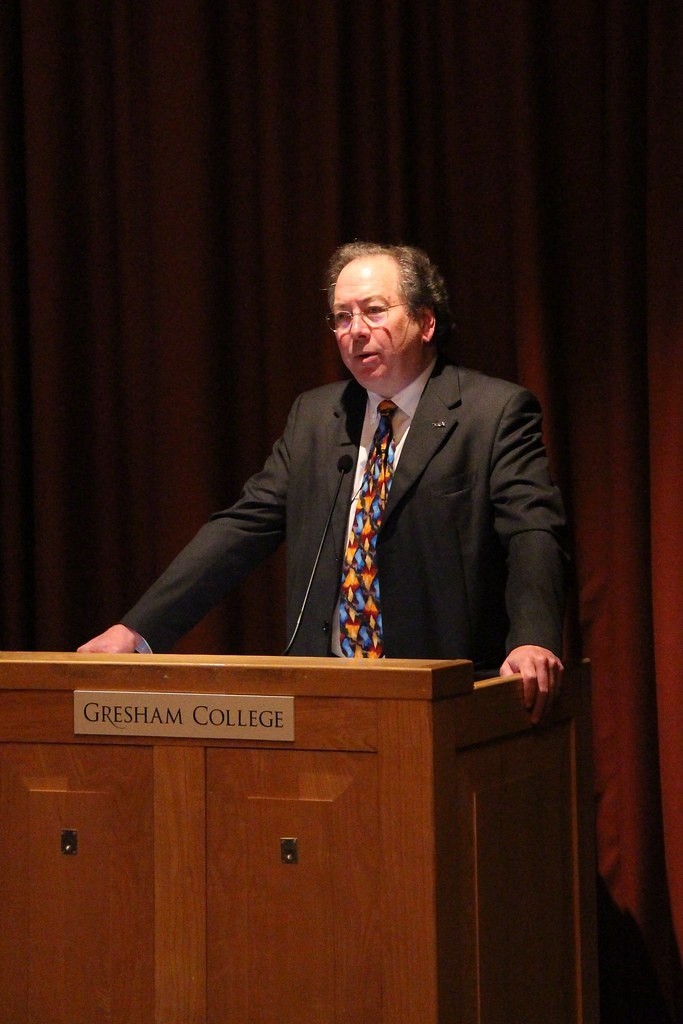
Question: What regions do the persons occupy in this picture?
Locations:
[77,240,570,723]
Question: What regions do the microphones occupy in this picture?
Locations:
[282,456,353,655]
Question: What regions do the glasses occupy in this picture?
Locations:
[325,300,410,332]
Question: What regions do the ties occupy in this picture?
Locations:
[339,399,402,660]
